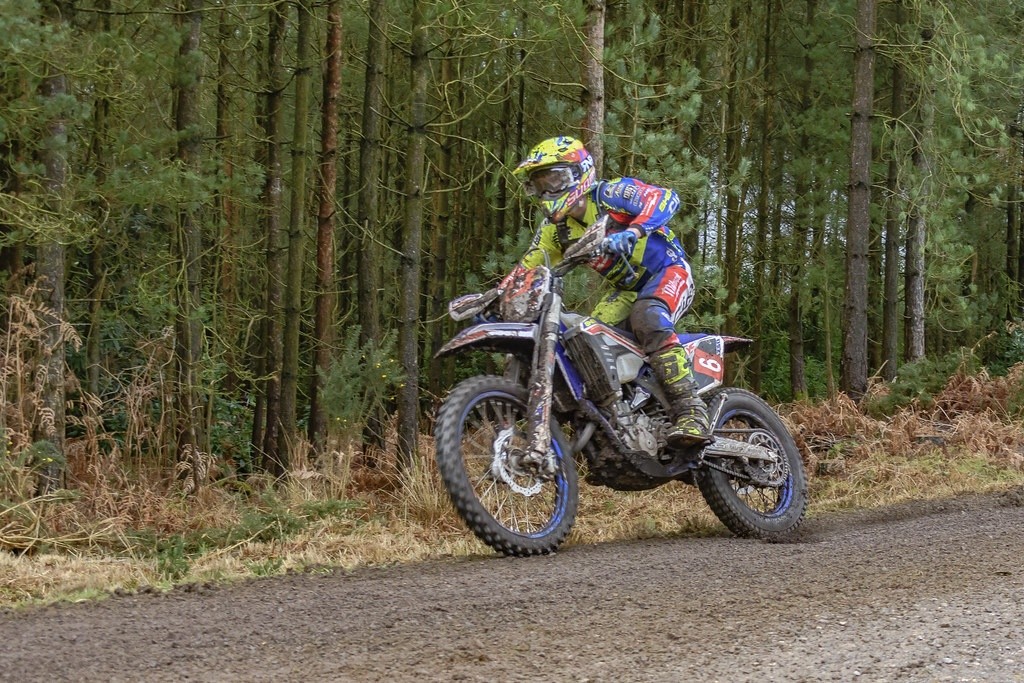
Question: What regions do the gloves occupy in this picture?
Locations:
[600,230,639,259]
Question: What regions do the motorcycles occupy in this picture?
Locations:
[432,214,809,557]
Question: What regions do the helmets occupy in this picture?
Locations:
[511,136,598,224]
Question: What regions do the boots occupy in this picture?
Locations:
[649,342,718,448]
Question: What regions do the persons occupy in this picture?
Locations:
[488,136,716,449]
